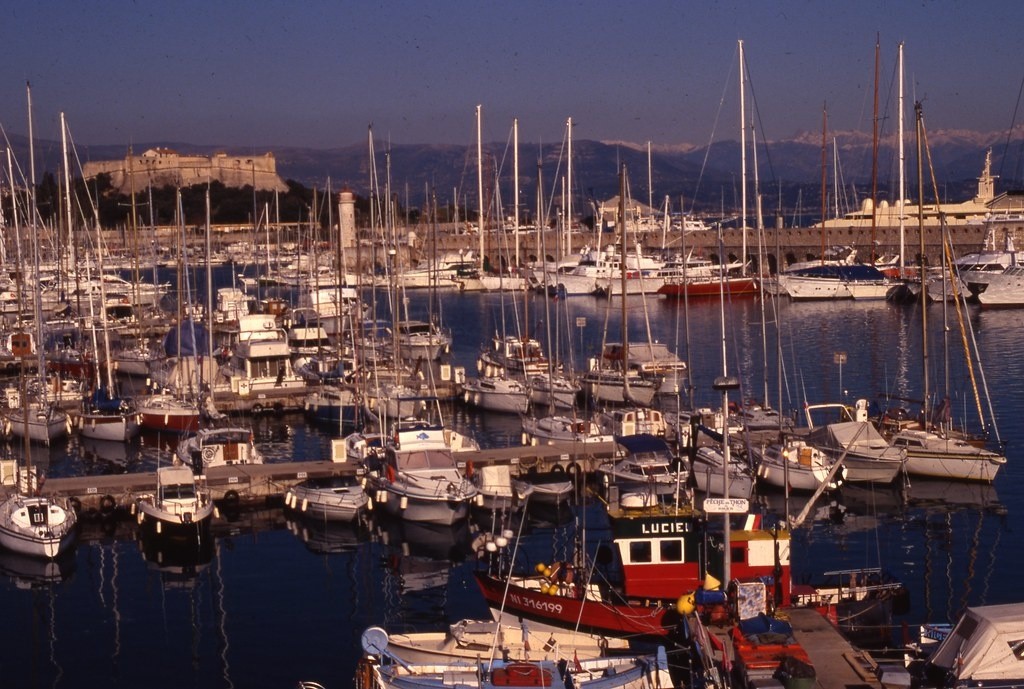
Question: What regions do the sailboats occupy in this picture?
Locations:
[0,40,1024,689]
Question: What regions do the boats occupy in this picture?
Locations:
[134,523,218,593]
[808,146,1023,224]
[268,471,373,525]
[977,265,1024,308]
[130,463,217,541]
[284,509,375,565]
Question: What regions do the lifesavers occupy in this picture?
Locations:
[224,490,239,505]
[549,562,573,587]
[566,462,582,474]
[386,464,397,484]
[100,495,115,514]
[252,403,263,414]
[67,497,81,512]
[551,464,564,473]
